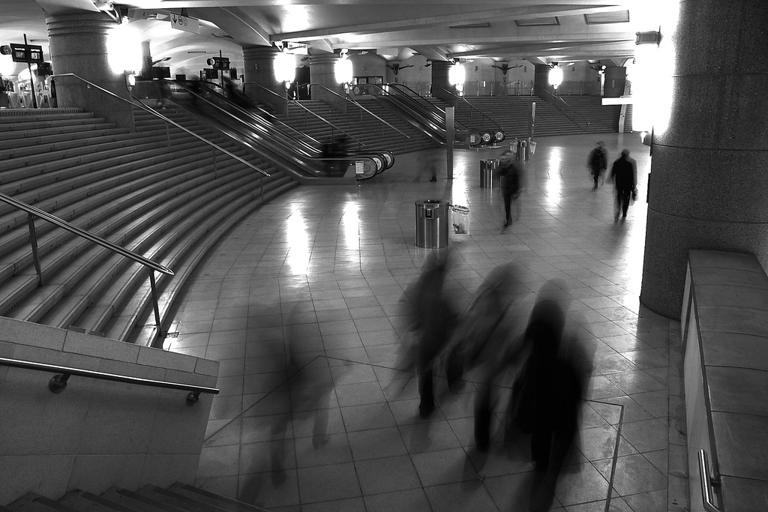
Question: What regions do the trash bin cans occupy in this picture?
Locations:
[517,141,526,161]
[415,199,470,249]
[480,158,494,188]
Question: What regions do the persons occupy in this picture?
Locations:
[642,130,651,145]
[605,150,637,224]
[492,151,520,227]
[587,140,604,192]
[257,251,595,511]
